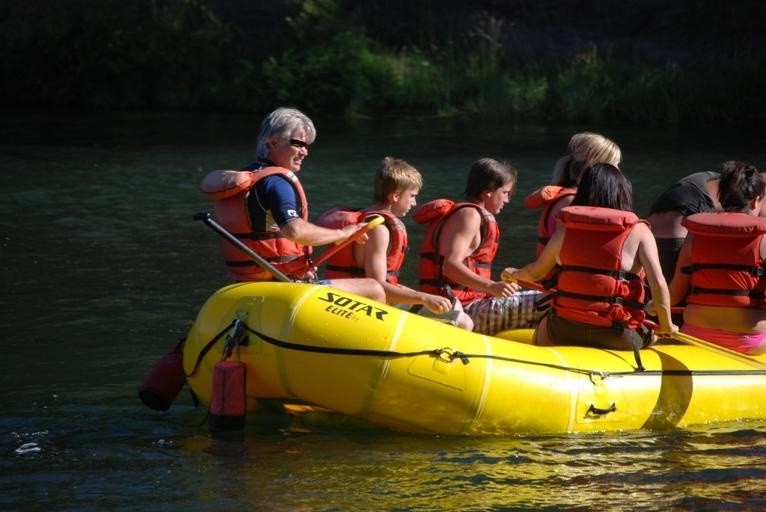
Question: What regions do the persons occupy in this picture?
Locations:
[498,163,680,351]
[645,159,766,357]
[200,105,386,305]
[315,157,475,333]
[520,132,622,289]
[412,158,549,338]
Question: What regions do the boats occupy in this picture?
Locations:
[185,282,762,436]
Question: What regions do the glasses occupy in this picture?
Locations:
[283,137,308,151]
